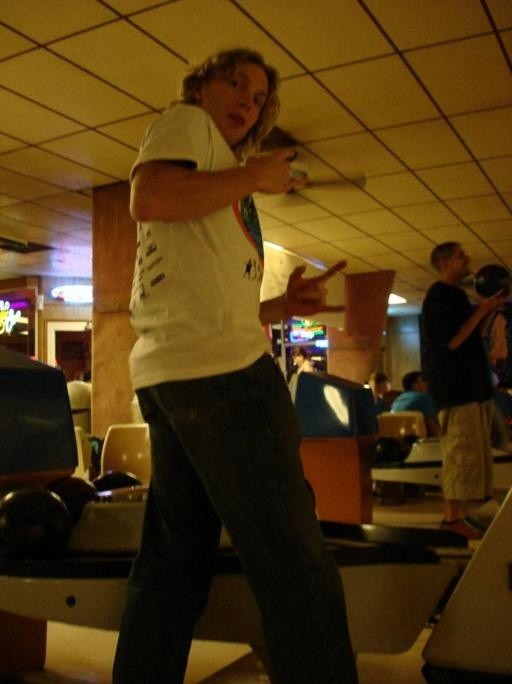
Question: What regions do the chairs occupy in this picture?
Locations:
[375,407,427,442]
[72,423,153,503]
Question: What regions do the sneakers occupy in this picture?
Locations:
[439,515,489,540]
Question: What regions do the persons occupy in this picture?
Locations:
[419,240,512,539]
[291,347,317,375]
[369,370,444,496]
[112,49,358,683]
